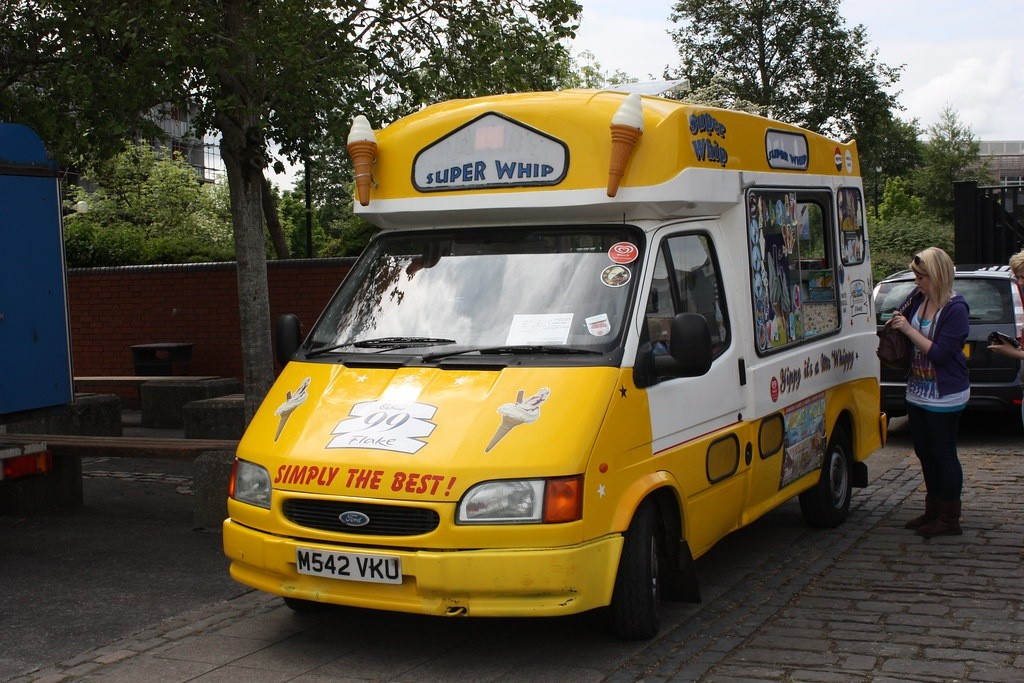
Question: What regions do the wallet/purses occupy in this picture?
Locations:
[987,331,1020,348]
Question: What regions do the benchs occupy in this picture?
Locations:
[0,433,242,534]
[74,374,245,439]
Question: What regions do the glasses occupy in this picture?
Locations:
[914,255,925,267]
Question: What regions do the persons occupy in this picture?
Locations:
[988,249,1024,422]
[876,247,970,535]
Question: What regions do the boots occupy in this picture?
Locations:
[905,494,963,536]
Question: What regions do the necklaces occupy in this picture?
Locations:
[919,295,939,341]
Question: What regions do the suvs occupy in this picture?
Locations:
[873,264,1024,436]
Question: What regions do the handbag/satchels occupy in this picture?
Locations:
[876,288,922,370]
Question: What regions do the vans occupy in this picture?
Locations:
[223,79,888,641]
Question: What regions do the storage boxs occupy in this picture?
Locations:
[808,269,834,299]
